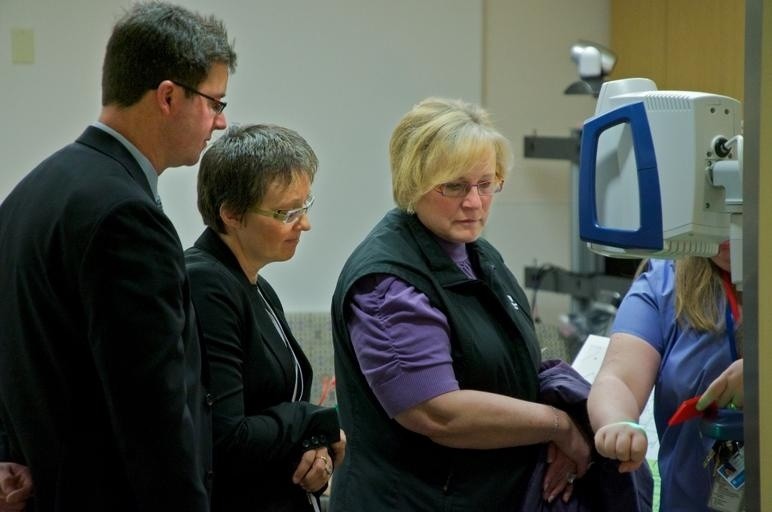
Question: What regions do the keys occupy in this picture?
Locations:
[701,440,739,478]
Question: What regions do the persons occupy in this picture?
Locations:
[183,123,347,512]
[587,239,745,511]
[332,96,592,512]
[1,3,237,512]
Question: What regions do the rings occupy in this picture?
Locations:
[727,404,735,409]
[325,463,333,474]
[587,461,594,469]
[316,455,327,462]
[567,473,575,483]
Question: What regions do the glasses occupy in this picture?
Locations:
[244,194,315,223]
[428,176,504,201]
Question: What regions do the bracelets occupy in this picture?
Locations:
[543,405,560,444]
